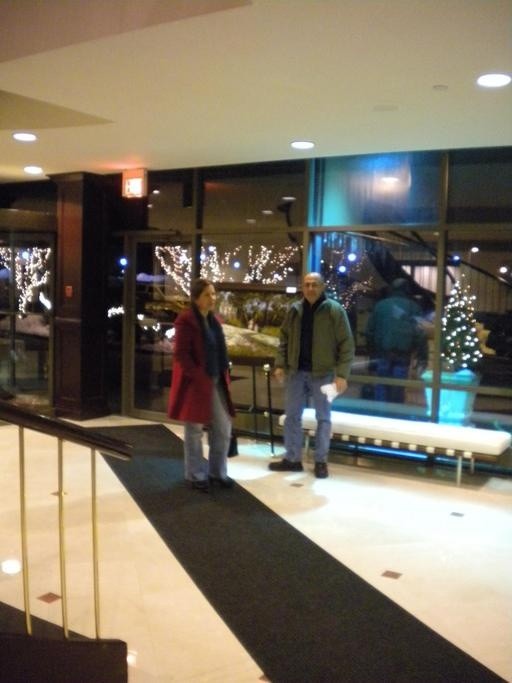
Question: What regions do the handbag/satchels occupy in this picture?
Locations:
[208,427,237,457]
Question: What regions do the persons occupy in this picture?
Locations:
[167,278,239,493]
[268,271,355,478]
[362,277,429,403]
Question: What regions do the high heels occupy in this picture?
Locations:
[192,481,209,489]
[208,475,236,488]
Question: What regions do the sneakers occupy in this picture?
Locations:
[269,459,302,471]
[314,461,327,477]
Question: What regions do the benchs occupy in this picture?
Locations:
[278,402,512,486]
